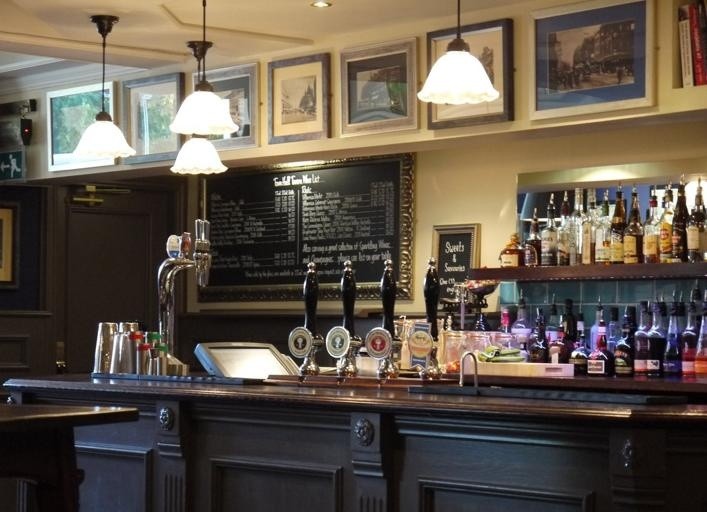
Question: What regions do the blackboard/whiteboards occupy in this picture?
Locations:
[434,224,477,298]
[196,151,415,302]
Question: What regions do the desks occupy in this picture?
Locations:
[0,403,139,510]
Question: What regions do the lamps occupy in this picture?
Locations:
[417,1,500,104]
[169,0,240,136]
[70,12,135,158]
[170,39,228,174]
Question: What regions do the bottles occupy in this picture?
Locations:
[501,171,707,265]
[498,277,707,376]
[130,326,168,377]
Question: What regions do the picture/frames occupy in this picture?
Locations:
[526,1,657,121]
[338,38,417,138]
[424,18,515,128]
[47,82,117,173]
[192,61,259,150]
[431,222,482,283]
[0,200,21,290]
[120,72,183,164]
[264,52,332,143]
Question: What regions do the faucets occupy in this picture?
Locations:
[157,220,215,356]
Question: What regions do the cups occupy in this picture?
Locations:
[109,330,132,374]
[93,321,118,373]
[119,321,138,333]
